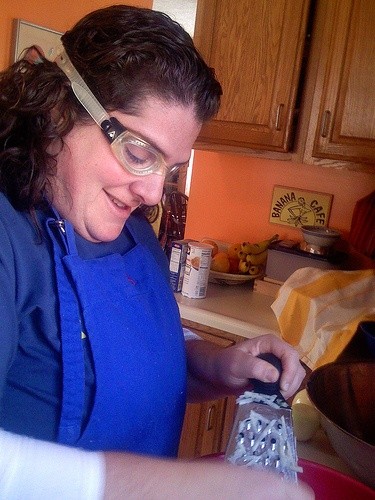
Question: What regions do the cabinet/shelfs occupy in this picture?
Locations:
[175,317,250,459]
[191,0,375,173]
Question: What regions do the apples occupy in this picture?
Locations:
[200,239,243,274]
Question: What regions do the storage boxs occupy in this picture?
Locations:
[252,276,285,298]
[263,239,350,283]
[169,239,199,293]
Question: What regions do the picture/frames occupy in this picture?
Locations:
[10,18,65,73]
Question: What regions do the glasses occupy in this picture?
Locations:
[41,33,189,179]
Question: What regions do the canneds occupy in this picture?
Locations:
[167,240,214,300]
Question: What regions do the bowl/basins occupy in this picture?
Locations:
[360,322,375,358]
[305,358,375,488]
[208,270,264,285]
[187,452,375,500]
[301,226,341,247]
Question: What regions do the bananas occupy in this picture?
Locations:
[237,233,279,275]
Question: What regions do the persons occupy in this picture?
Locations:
[0,4,315,500]
